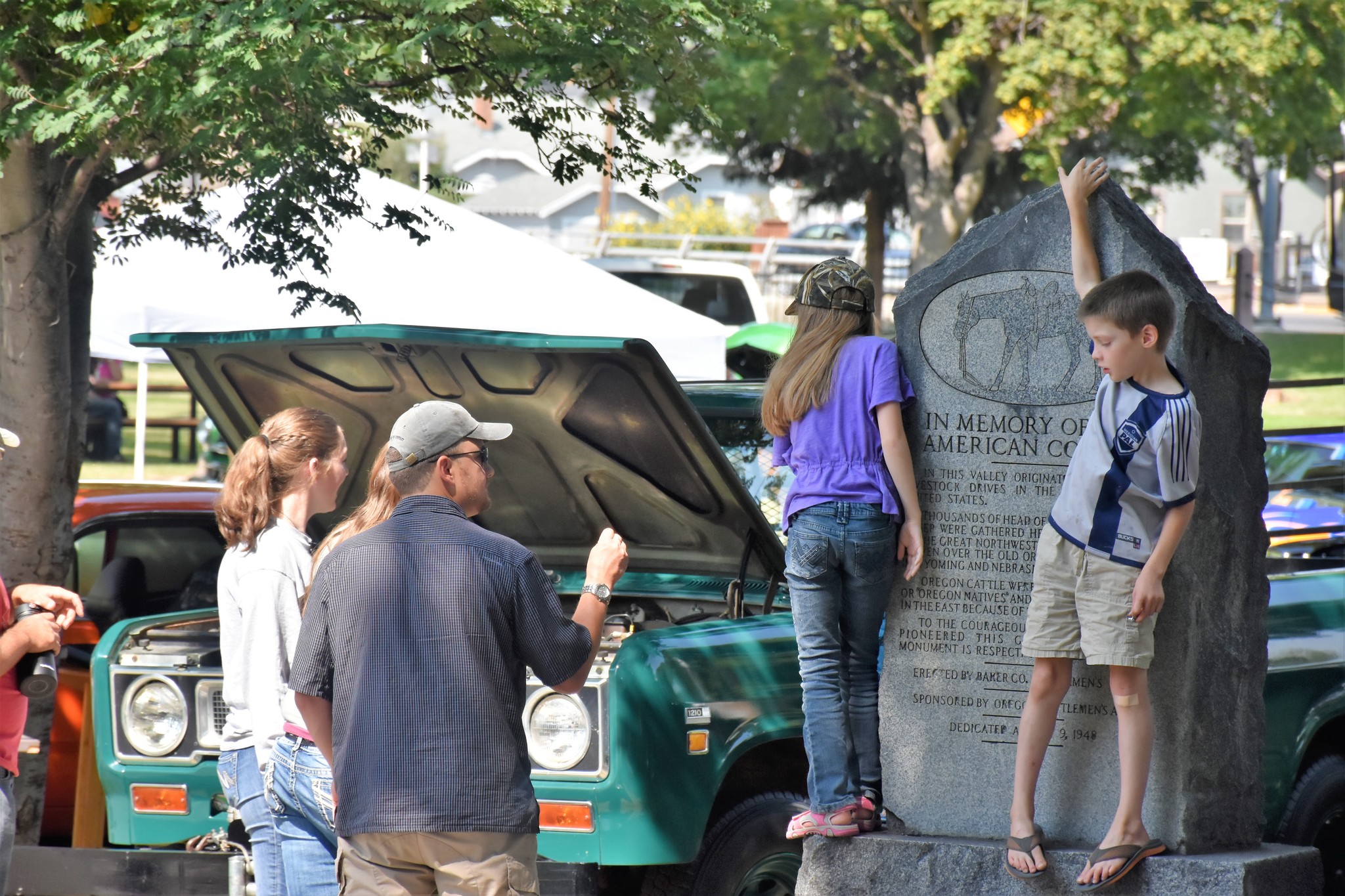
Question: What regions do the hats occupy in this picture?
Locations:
[387,399,512,472]
[784,255,876,316]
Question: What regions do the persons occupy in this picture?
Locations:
[0,425,86,896]
[218,405,346,896]
[264,439,397,896]
[1004,157,1203,894]
[86,381,127,462]
[291,396,628,896]
[761,258,923,839]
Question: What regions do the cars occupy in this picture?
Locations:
[45,481,237,851]
[588,257,764,325]
[83,382,128,459]
[97,330,1343,891]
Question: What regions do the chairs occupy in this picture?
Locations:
[73,555,150,623]
[163,553,223,610]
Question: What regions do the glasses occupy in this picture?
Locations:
[429,447,489,465]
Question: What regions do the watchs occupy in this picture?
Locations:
[580,584,612,606]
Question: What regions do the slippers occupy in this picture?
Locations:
[1004,824,1048,880]
[1075,838,1167,892]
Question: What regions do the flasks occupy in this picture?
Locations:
[12,602,58,700]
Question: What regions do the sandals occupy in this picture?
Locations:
[857,796,883,830]
[785,802,858,839]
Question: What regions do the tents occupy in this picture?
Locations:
[93,162,739,483]
